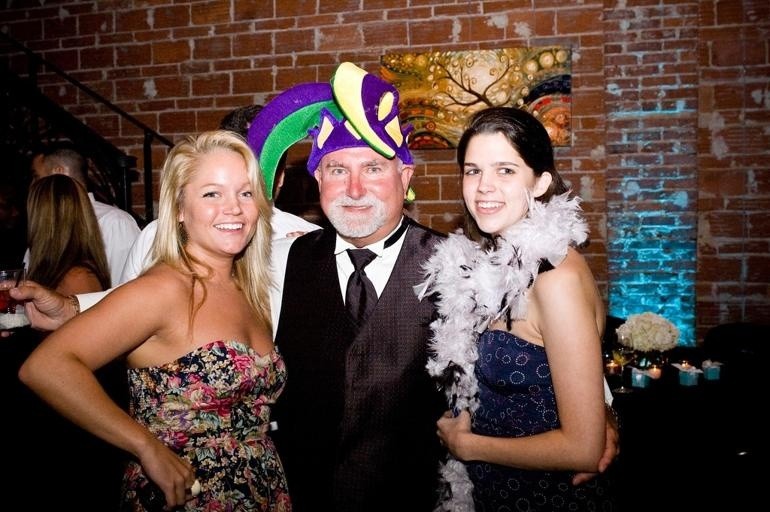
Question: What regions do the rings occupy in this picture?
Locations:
[186,478,202,498]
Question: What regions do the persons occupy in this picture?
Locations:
[412,106,607,512]
[0,268,135,333]
[23,174,111,297]
[22,140,141,287]
[18,128,293,512]
[1,59,621,512]
[118,103,324,288]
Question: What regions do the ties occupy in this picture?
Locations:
[344,216,408,342]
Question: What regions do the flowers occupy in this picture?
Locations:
[615,311,679,353]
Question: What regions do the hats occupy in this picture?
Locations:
[248,62,412,201]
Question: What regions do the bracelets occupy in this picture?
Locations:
[67,294,80,317]
[604,402,620,425]
